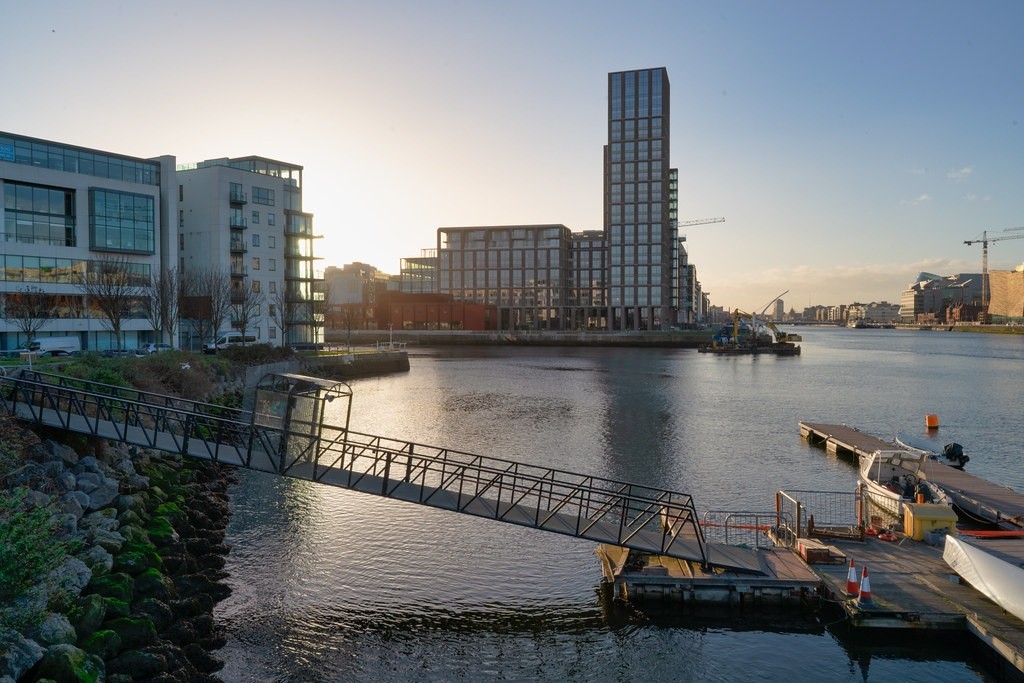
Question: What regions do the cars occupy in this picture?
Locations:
[33,346,147,359]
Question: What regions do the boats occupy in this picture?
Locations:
[859,449,953,517]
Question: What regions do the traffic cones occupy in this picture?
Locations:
[851,566,878,610]
[840,558,860,598]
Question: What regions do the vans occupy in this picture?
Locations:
[13,336,81,357]
[202,330,257,355]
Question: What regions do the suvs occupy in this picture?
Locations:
[143,343,183,354]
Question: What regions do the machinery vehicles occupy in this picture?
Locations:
[733,307,802,354]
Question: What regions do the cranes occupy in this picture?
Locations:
[962,231,1023,323]
